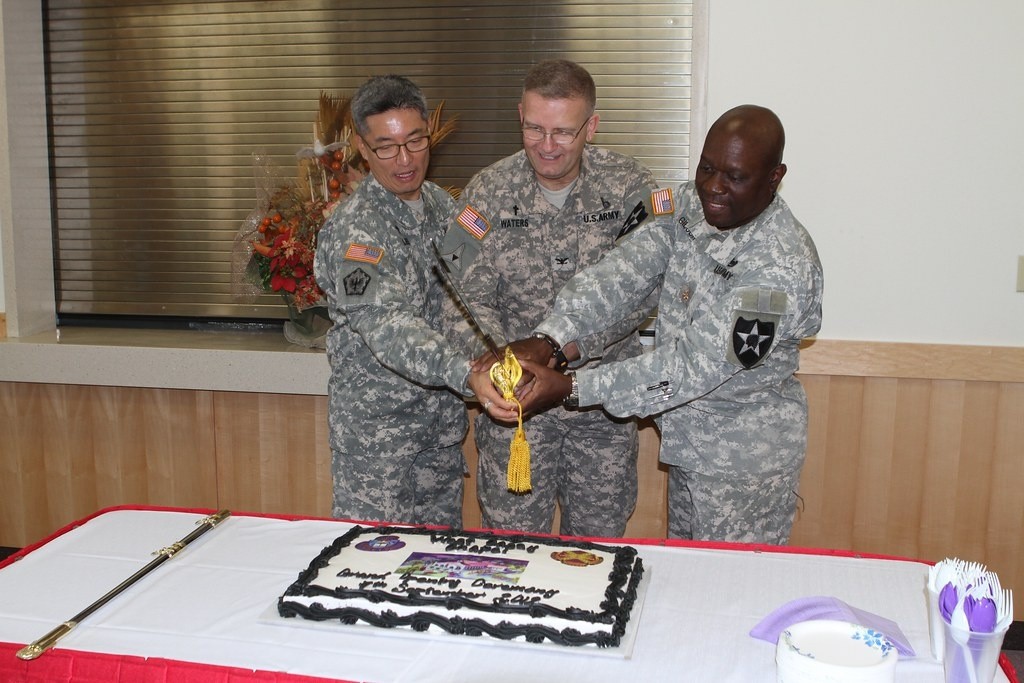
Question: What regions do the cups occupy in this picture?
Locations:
[941,617,1010,683]
[926,583,944,662]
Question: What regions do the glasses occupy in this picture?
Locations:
[521,115,592,145]
[356,120,430,160]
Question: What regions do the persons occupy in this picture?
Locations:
[437,59,660,538]
[311,72,520,530]
[468,104,825,546]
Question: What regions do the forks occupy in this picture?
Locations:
[928,558,1014,633]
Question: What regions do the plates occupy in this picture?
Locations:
[774,620,897,683]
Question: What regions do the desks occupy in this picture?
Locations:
[0,504,1021,683]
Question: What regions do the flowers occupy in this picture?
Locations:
[250,138,370,336]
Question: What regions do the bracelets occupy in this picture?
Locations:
[532,332,557,357]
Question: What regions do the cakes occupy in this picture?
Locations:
[277,526,644,648]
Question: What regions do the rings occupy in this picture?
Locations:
[483,398,492,412]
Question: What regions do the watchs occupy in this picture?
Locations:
[551,350,567,373]
[562,372,580,412]
